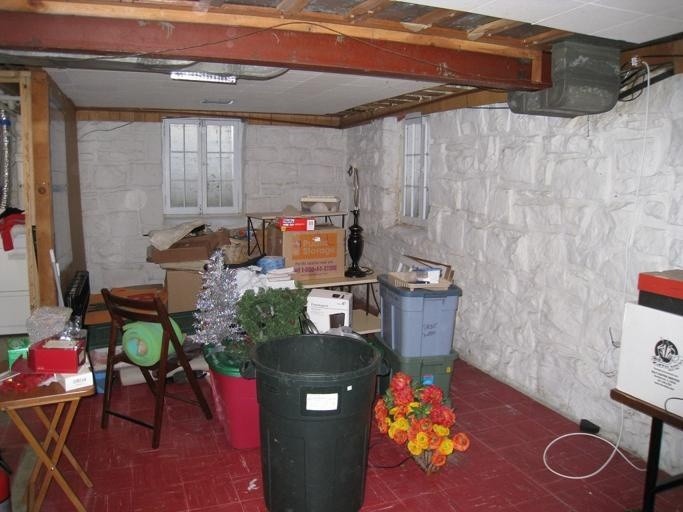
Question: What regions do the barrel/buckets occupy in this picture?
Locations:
[121,314,183,367]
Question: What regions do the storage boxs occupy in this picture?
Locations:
[265,220,347,281]
[369,273,465,401]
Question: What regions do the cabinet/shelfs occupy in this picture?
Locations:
[289,273,383,340]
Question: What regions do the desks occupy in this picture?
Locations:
[0,349,99,510]
[246,212,348,258]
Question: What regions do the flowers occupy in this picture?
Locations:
[375,371,470,475]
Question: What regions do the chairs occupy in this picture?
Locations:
[92,290,214,449]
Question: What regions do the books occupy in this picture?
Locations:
[264,266,295,288]
